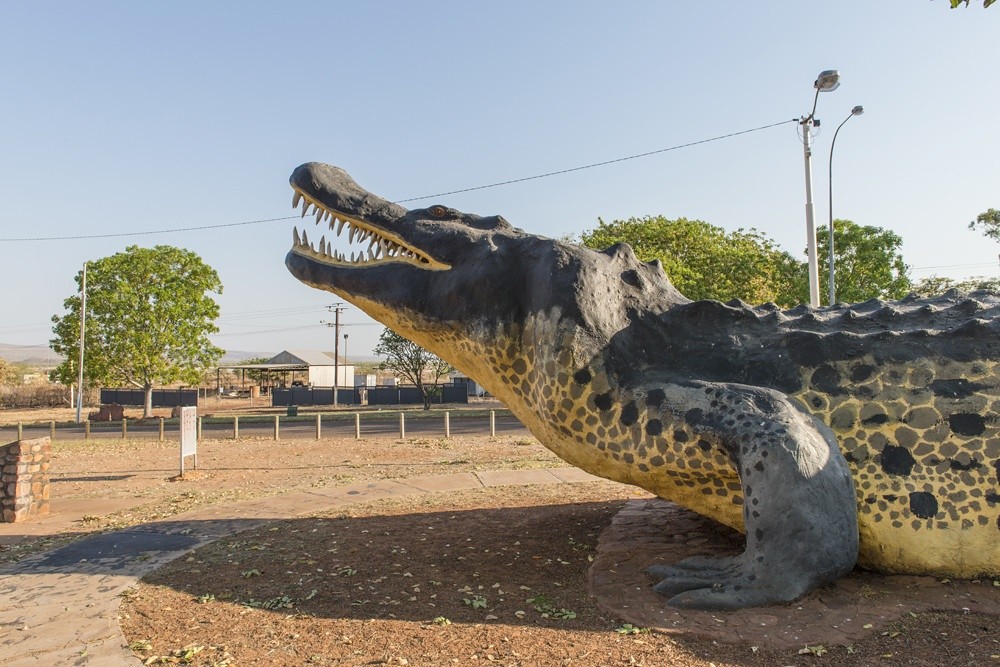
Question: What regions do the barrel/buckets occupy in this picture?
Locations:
[249,385,260,398]
[287,407,297,416]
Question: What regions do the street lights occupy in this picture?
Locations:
[798,69,840,310]
[343,333,349,389]
[829,105,866,306]
